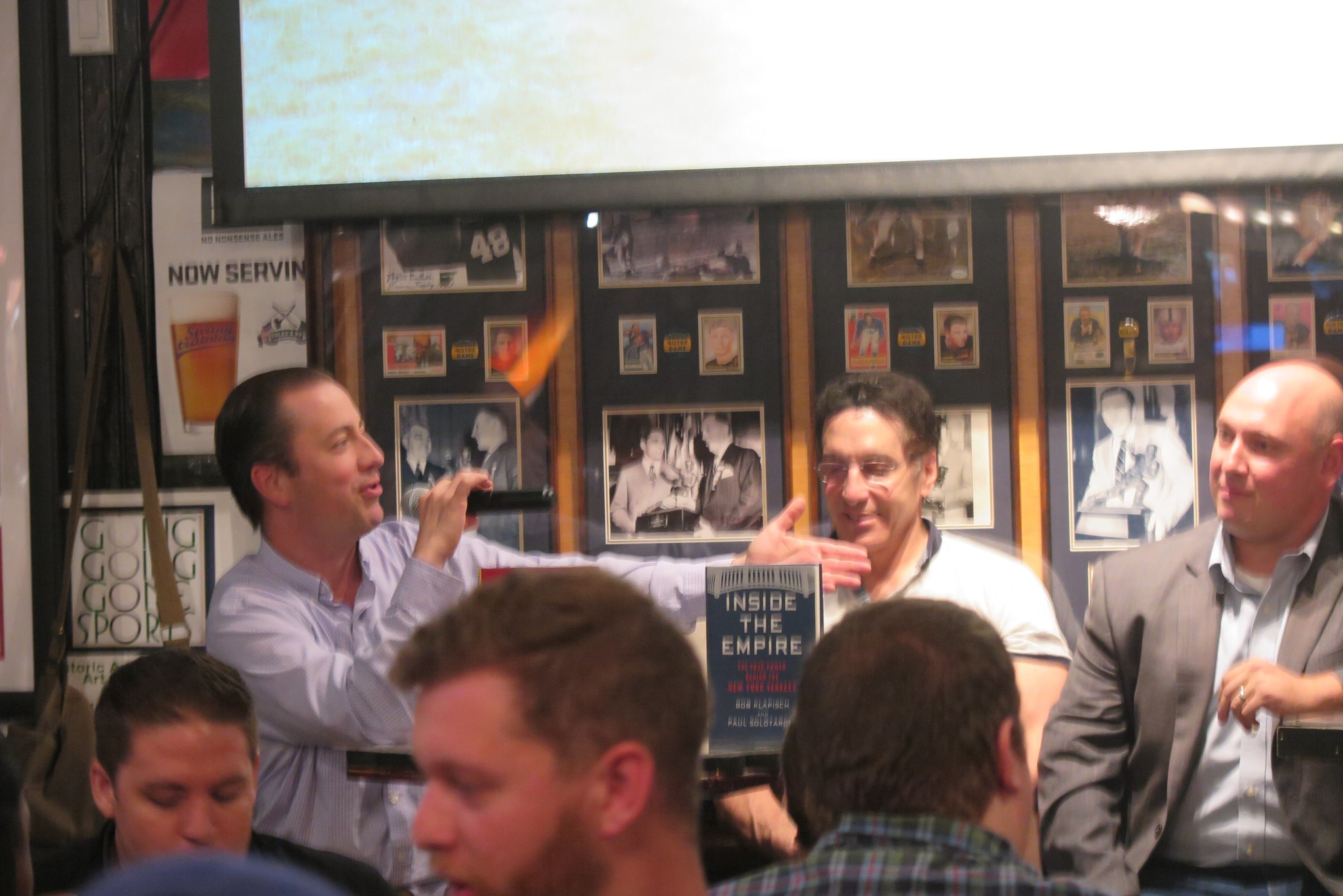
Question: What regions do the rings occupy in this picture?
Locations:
[1239,686,1247,702]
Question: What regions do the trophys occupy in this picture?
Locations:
[666,458,697,509]
[1075,443,1161,541]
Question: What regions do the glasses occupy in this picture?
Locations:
[811,452,911,485]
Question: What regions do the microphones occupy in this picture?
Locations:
[403,484,554,520]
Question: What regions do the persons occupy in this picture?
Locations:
[1154,305,1189,359]
[1068,305,1107,365]
[854,195,953,270]
[383,564,711,895]
[1074,386,1195,543]
[699,598,1106,895]
[601,211,755,282]
[35,647,396,896]
[922,417,972,523]
[716,372,1074,867]
[205,366,873,895]
[401,405,520,552]
[1273,304,1309,352]
[1038,359,1343,895]
[609,413,762,533]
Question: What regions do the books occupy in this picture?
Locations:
[706,561,824,741]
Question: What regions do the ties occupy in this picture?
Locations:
[648,465,656,488]
[415,463,422,480]
[1115,440,1128,502]
